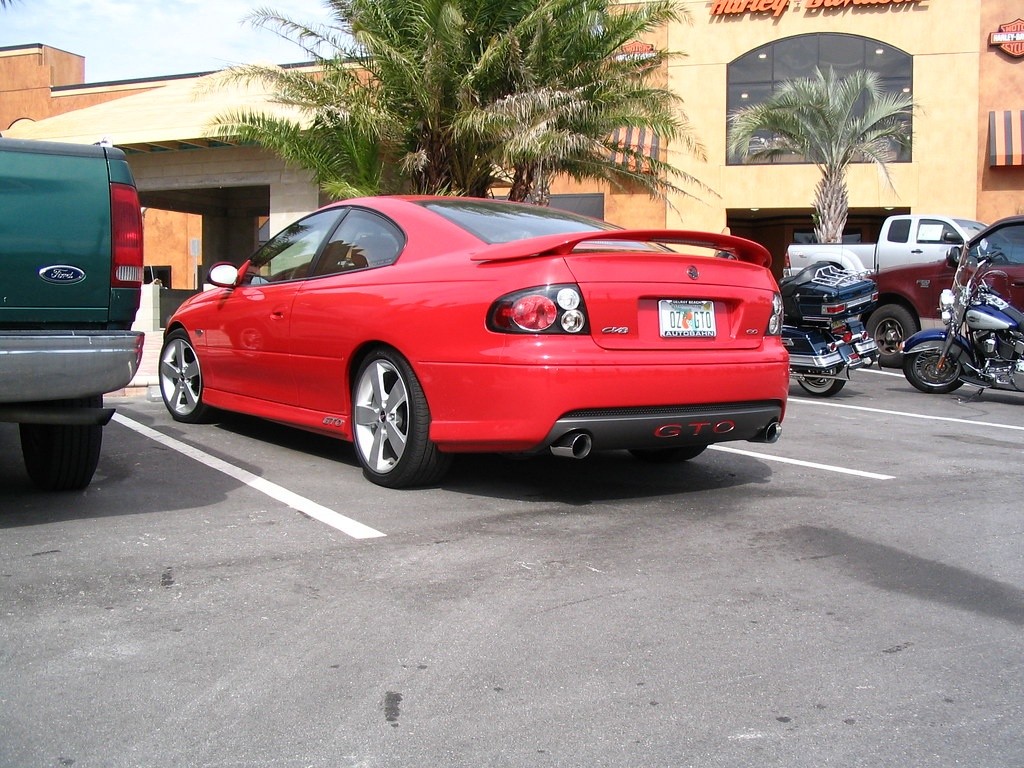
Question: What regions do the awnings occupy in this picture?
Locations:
[989,111,1024,168]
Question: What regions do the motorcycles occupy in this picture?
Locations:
[715,228,878,396]
[898,238,1024,402]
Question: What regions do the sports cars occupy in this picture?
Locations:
[157,194,791,487]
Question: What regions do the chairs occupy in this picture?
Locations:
[350,237,397,271]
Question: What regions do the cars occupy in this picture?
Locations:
[860,216,1023,369]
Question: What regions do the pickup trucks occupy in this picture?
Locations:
[0,136,148,494]
[782,216,1015,291]
